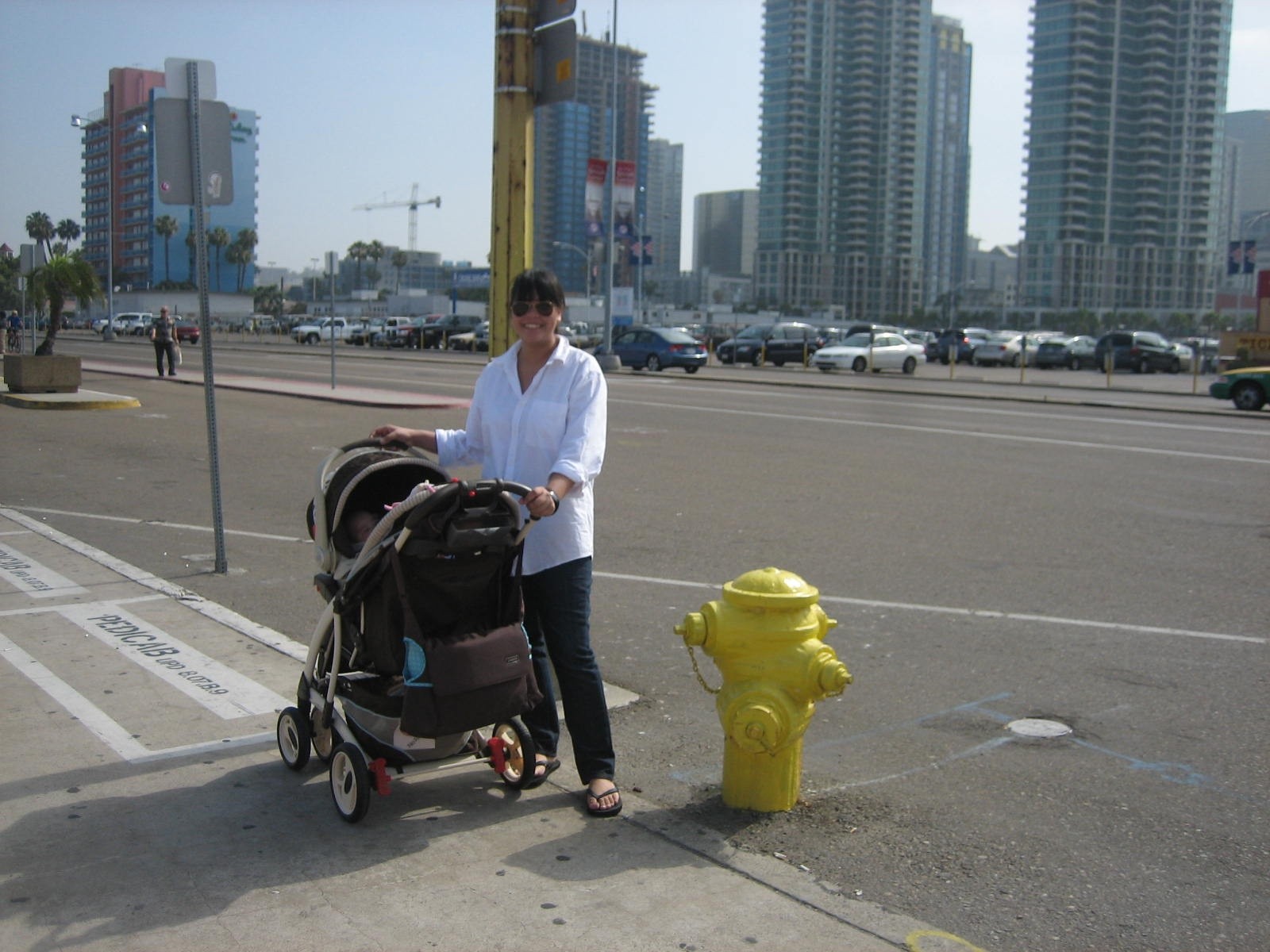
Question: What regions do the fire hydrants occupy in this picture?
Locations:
[684,574,860,821]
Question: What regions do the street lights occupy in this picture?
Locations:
[69,114,147,343]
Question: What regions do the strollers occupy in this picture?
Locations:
[275,437,555,823]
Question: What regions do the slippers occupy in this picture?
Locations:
[586,782,622,817]
[517,759,561,787]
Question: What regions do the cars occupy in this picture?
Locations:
[807,325,1097,367]
[1096,331,1195,377]
[811,332,929,374]
[93,312,183,336]
[714,321,825,368]
[149,319,201,345]
[291,312,489,353]
[591,325,713,376]
[1208,368,1270,413]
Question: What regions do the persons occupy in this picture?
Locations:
[345,503,384,558]
[6,310,24,340]
[371,267,625,818]
[150,306,178,377]
[0,311,8,354]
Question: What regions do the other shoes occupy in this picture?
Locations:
[169,373,177,376]
[159,373,164,377]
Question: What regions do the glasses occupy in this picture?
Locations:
[510,300,554,317]
[160,310,168,313]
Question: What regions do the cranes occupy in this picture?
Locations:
[350,194,443,251]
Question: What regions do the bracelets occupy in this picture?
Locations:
[544,487,561,516]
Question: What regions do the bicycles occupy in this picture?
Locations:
[5,331,22,354]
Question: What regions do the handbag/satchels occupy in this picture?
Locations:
[174,346,183,366]
